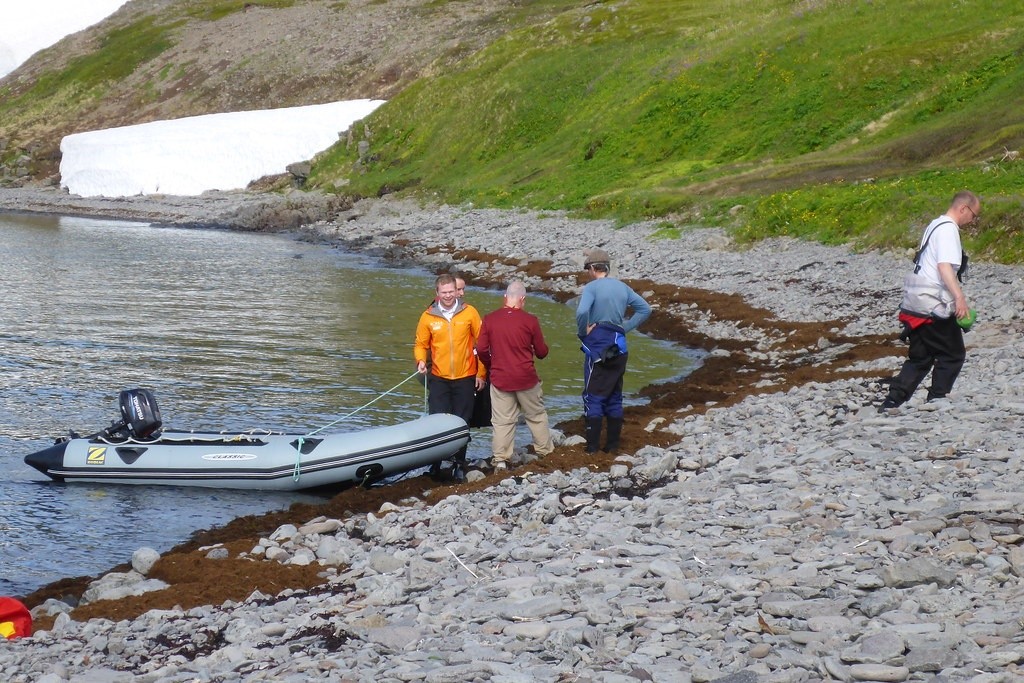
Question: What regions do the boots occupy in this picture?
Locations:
[584,417,624,453]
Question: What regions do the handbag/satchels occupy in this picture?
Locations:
[469,373,492,428]
[899,272,956,318]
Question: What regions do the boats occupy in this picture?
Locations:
[23,387,470,493]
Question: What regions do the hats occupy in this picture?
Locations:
[584,249,611,270]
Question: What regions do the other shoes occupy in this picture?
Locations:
[877,399,898,413]
[453,468,464,480]
[494,462,507,475]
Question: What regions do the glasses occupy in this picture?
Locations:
[966,206,979,222]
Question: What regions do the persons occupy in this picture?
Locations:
[878,192,981,412]
[414,273,488,483]
[476,282,555,474]
[577,250,652,454]
